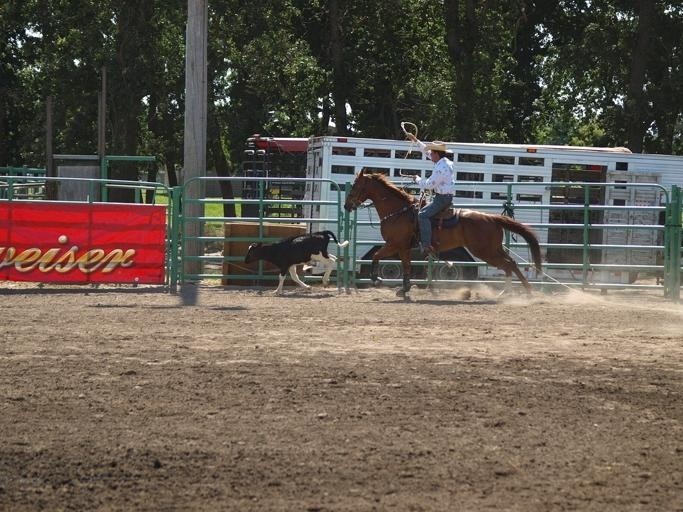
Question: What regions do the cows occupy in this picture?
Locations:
[245,231,349,296]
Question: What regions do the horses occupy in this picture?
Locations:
[343,167,542,297]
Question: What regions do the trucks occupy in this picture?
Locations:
[303,135,683,290]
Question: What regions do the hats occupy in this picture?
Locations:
[425,141,446,151]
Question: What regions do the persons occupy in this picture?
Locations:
[404,133,457,252]
